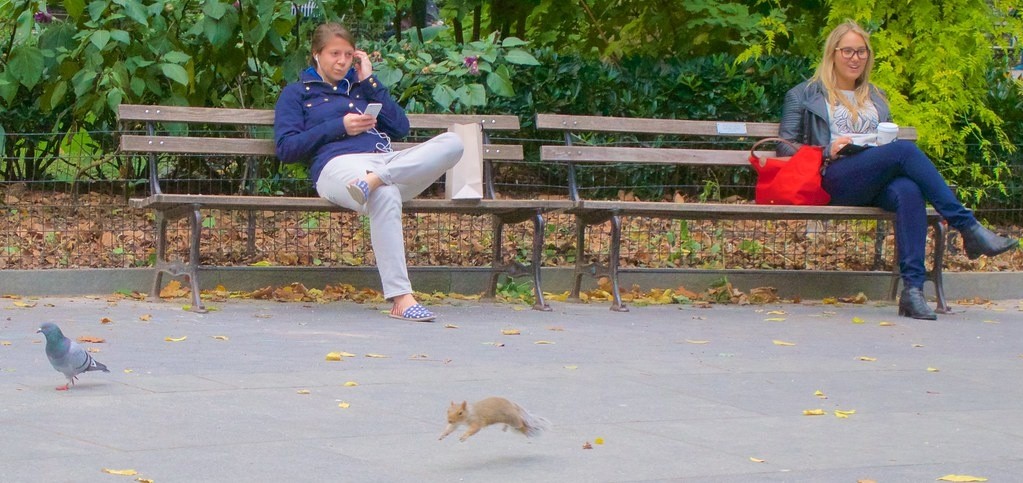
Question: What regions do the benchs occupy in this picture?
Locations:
[116,103,577,315]
[535,113,956,315]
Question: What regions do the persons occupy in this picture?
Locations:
[776,19,1018,320]
[273,22,465,321]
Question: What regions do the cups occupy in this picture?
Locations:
[876,122,899,146]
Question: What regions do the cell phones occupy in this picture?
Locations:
[364,104,382,119]
[836,143,878,157]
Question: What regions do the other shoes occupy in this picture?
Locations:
[346,177,369,206]
[389,303,436,320]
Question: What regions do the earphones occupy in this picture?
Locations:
[315,54,318,60]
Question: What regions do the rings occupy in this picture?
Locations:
[365,56,367,59]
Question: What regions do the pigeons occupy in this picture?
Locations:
[36,322,110,390]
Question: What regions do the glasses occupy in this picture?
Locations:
[835,47,870,59]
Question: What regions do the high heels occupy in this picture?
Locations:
[959,219,1018,261]
[898,281,939,321]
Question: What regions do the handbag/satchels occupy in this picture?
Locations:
[749,136,832,206]
